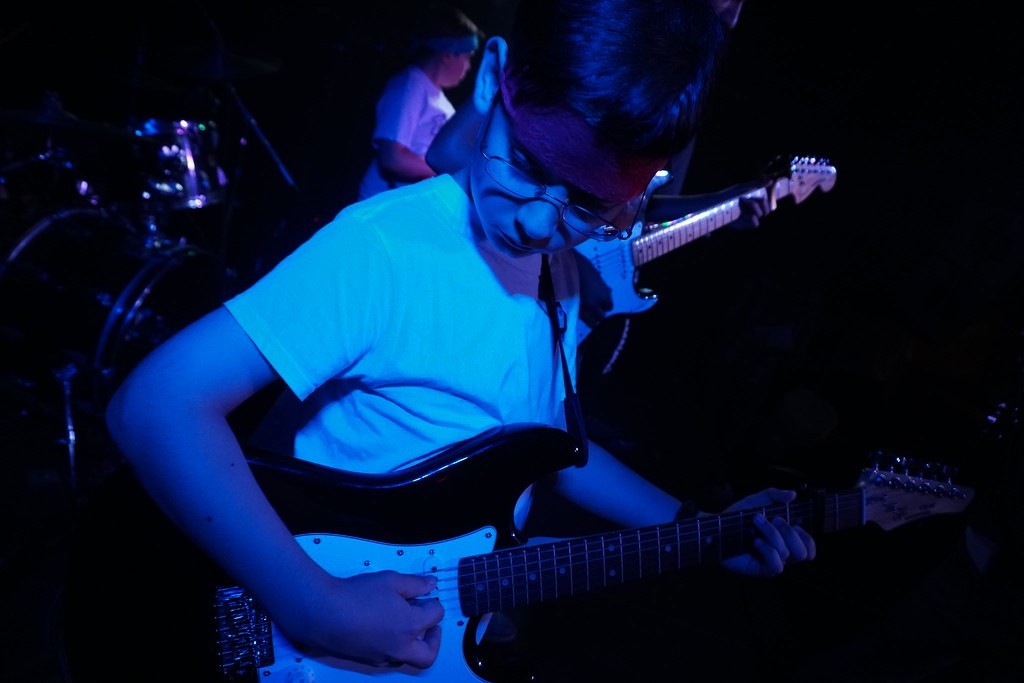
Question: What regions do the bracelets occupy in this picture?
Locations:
[673,500,699,524]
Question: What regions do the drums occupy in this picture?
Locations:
[2,103,244,452]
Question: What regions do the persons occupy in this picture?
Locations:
[104,1,816,683]
[572,1,771,388]
[355,7,479,200]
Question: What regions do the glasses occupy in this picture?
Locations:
[479,86,655,242]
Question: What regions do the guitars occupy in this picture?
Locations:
[50,420,987,680]
[575,152,837,319]
